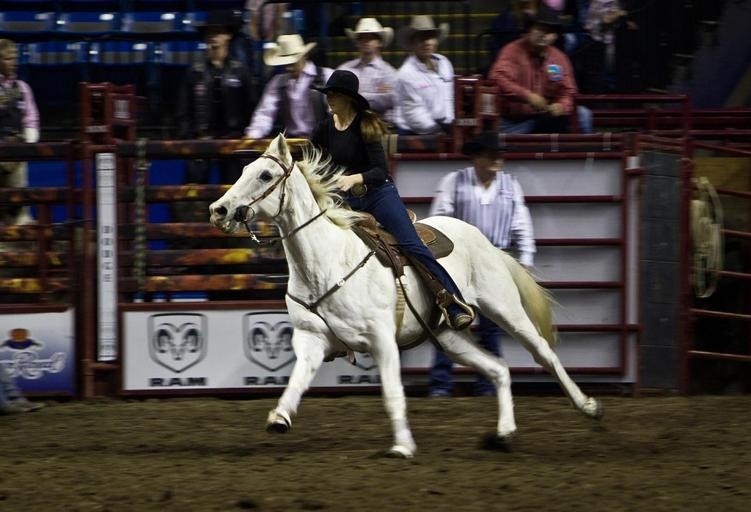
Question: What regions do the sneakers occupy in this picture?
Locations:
[452,312,472,330]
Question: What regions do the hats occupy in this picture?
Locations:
[344,18,394,48]
[461,130,518,155]
[310,70,369,110]
[522,6,578,32]
[401,16,440,42]
[263,33,317,66]
[191,8,246,27]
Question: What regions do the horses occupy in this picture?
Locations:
[207,129,604,460]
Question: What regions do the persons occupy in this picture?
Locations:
[0,38,43,255]
[307,70,475,329]
[484,12,600,152]
[389,12,458,151]
[493,0,751,110]
[424,132,537,400]
[333,16,397,132]
[175,10,252,182]
[241,34,337,139]
[239,1,293,88]
